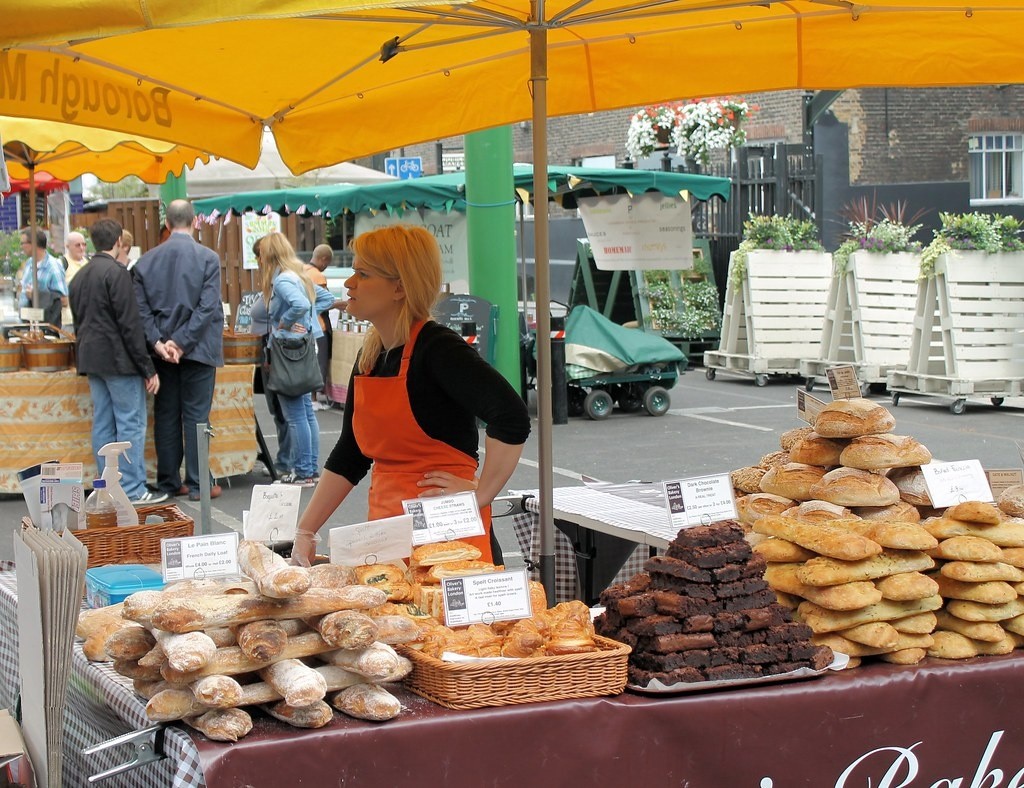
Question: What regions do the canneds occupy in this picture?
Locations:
[338,318,372,333]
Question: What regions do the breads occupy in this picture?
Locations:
[596,517,835,688]
[350,541,601,659]
[74,540,420,741]
[728,396,1024,669]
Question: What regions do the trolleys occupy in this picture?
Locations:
[529,298,679,423]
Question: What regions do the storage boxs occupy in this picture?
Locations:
[82,564,162,610]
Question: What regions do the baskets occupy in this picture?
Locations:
[393,636,632,710]
[56,503,195,569]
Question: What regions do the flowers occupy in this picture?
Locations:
[638,252,722,344]
[626,102,675,159]
[670,98,755,166]
[917,209,1024,278]
[731,211,823,294]
[833,190,921,272]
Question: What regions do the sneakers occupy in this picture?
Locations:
[273,468,320,488]
[131,491,169,505]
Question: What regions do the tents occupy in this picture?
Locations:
[148,127,402,200]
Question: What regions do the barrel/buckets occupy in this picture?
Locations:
[0,343,22,372]
[22,341,72,370]
[224,335,264,364]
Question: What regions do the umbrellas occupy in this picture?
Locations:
[0,115,220,309]
[0,0,1024,610]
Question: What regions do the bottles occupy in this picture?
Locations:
[338,318,371,334]
[84,478,117,531]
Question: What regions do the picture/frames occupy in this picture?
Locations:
[717,105,746,138]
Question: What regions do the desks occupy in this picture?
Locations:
[510,473,733,605]
[0,562,1024,788]
[0,361,261,498]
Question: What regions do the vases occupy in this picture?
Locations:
[655,121,674,144]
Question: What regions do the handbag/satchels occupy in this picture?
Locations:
[253,366,264,394]
[266,328,325,399]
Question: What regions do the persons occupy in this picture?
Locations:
[18,227,69,339]
[259,233,335,488]
[68,219,169,505]
[133,199,226,500]
[115,229,138,271]
[250,237,291,476]
[288,225,531,571]
[303,244,347,411]
[58,231,91,339]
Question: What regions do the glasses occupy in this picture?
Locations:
[74,242,87,248]
[20,241,29,246]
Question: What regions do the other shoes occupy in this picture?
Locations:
[189,486,220,501]
[179,484,190,495]
[262,467,286,476]
[312,401,331,412]
